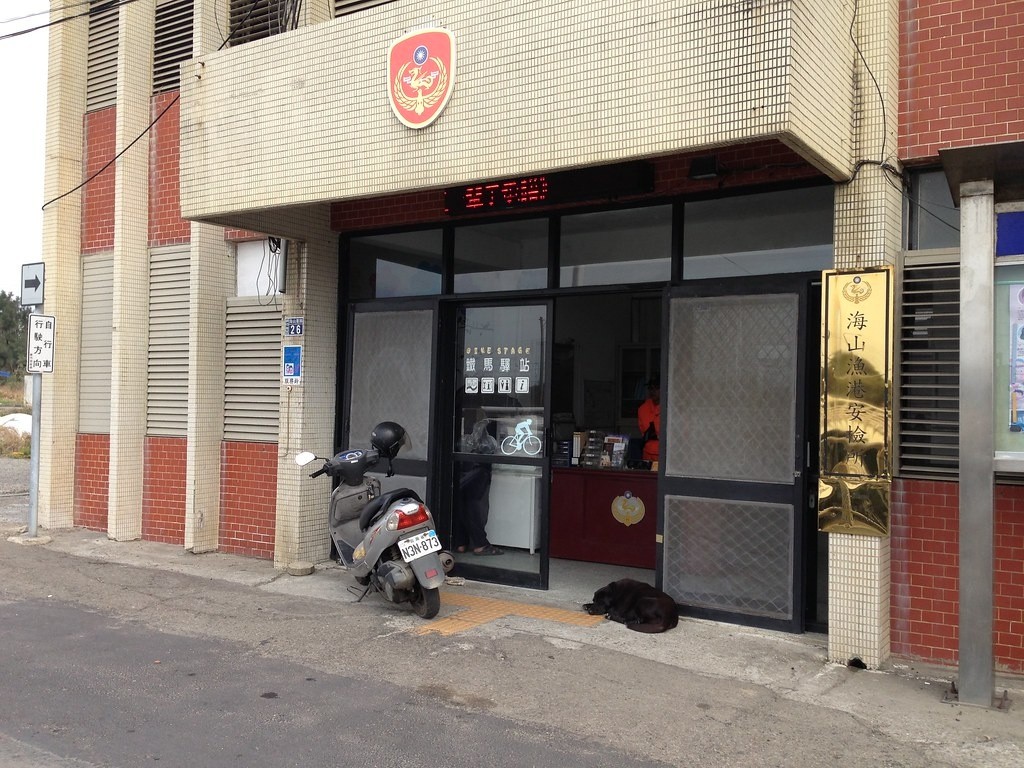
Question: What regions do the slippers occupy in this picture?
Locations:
[473,545,505,555]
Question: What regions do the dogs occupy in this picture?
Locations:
[582,578,679,634]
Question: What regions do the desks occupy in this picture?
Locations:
[548,465,660,570]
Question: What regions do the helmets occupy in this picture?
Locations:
[370,421,412,459]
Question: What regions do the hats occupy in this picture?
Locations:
[643,375,660,386]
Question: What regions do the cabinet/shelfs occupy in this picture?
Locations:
[612,340,663,440]
[485,414,547,556]
[552,349,583,454]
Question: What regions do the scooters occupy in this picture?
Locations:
[295,437,456,620]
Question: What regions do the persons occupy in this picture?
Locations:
[451,357,523,557]
[637,378,661,462]
